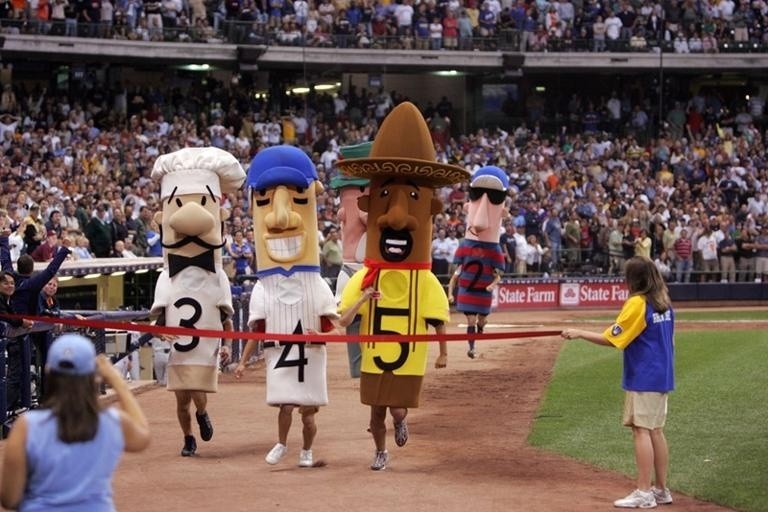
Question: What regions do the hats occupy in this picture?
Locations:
[48,332,97,374]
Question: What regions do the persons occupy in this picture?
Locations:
[446,165,507,360]
[1,62,256,295]
[114,319,171,387]
[1,0,768,52]
[421,92,768,284]
[151,146,246,457]
[558,256,676,508]
[329,141,373,382]
[1,228,72,440]
[235,144,338,466]
[1,334,151,511]
[336,102,471,471]
[238,81,400,268]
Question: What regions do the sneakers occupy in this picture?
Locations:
[614,489,657,508]
[394,422,409,446]
[371,450,388,471]
[196,411,213,441]
[652,485,673,504]
[468,349,476,358]
[299,449,312,466]
[182,436,197,456]
[265,443,287,464]
